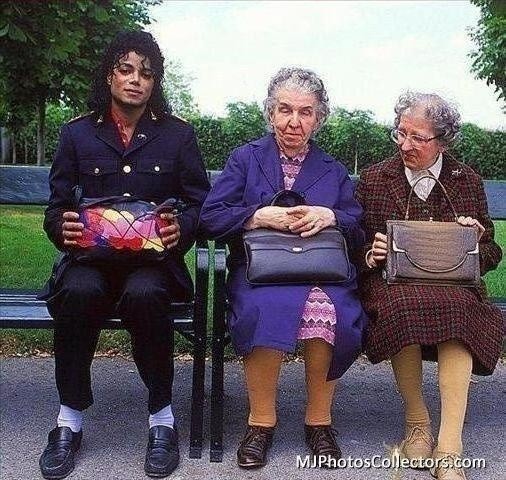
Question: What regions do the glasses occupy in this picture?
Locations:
[390,129,444,148]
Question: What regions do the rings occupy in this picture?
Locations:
[163,212,168,221]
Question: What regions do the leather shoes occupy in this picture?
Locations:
[303,423,341,468]
[404,423,432,464]
[39,426,83,480]
[143,425,180,477]
[430,446,466,480]
[237,425,273,468]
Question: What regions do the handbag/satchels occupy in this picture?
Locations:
[72,196,170,262]
[385,175,482,289]
[243,189,352,288]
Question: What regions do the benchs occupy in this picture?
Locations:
[1,165,205,461]
[200,169,505,464]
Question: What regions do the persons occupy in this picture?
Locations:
[195,65,370,471]
[351,85,504,480]
[34,26,213,480]
